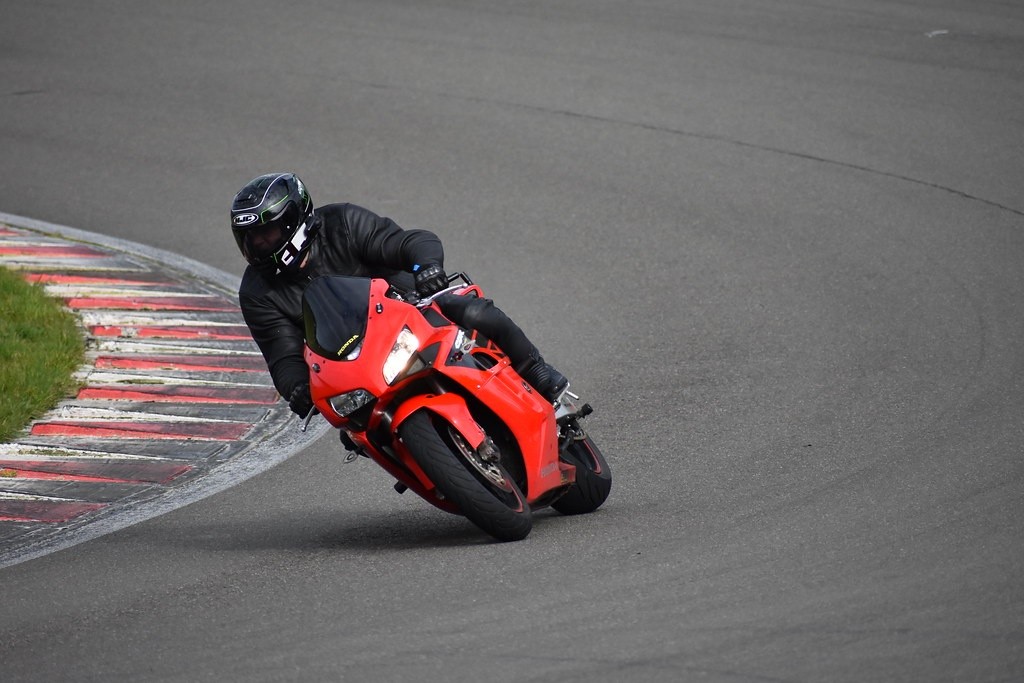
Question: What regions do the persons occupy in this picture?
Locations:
[229,173,568,458]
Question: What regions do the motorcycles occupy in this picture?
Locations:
[301,273,612,543]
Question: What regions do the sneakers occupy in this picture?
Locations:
[524,357,553,397]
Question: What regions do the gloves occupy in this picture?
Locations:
[289,385,321,419]
[414,262,449,298]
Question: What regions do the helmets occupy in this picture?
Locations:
[230,172,315,278]
